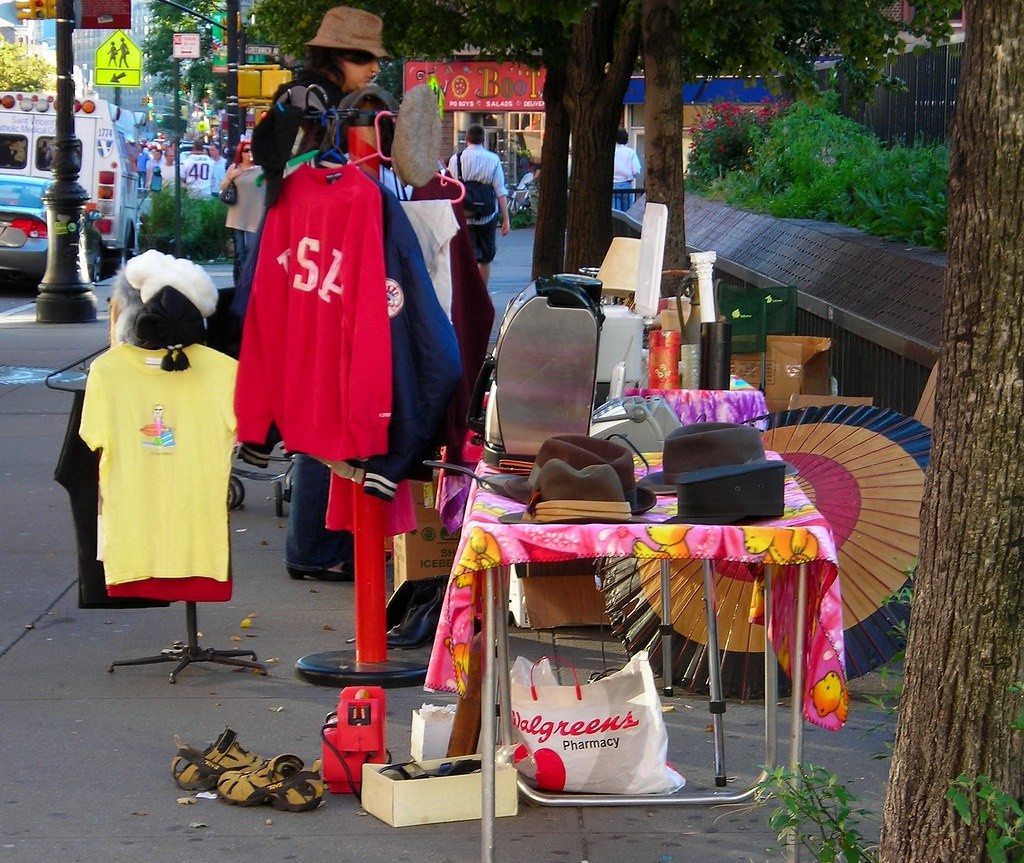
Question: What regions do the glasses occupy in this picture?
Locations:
[241,148,254,152]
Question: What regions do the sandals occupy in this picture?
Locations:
[378,759,482,779]
[217,754,324,812]
[170,725,267,791]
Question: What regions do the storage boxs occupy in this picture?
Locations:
[392,478,465,585]
[362,753,516,827]
[505,566,615,628]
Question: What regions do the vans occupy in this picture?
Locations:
[0,91,155,281]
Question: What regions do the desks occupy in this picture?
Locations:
[431,446,851,863]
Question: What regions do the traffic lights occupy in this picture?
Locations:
[148,98,152,107]
[14,0,35,20]
[178,88,186,99]
[255,109,270,126]
[31,0,48,20]
[141,97,147,106]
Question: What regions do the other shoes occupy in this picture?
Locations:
[288,562,353,581]
[386,579,447,648]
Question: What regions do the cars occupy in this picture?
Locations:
[0,172,105,285]
[151,138,210,165]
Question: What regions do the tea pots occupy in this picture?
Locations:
[677,251,725,341]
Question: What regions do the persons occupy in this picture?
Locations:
[219,140,258,286]
[209,144,228,196]
[272,6,390,109]
[181,138,214,198]
[136,143,175,185]
[448,123,511,285]
[613,126,641,212]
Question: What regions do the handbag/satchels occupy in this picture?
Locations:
[510,650,689,797]
[459,181,495,221]
[218,163,237,205]
[714,280,796,354]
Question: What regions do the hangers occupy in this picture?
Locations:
[256,85,466,203]
[44,340,113,391]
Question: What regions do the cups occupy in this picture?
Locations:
[649,330,679,390]
[700,321,731,390]
[681,344,700,389]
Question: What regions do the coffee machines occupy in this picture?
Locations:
[466,273,606,464]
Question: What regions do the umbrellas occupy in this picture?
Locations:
[600,403,931,702]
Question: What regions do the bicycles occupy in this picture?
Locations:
[506,182,539,216]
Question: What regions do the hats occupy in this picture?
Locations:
[503,435,657,516]
[390,84,441,186]
[636,422,799,495]
[660,464,786,524]
[498,458,663,525]
[304,5,389,57]
